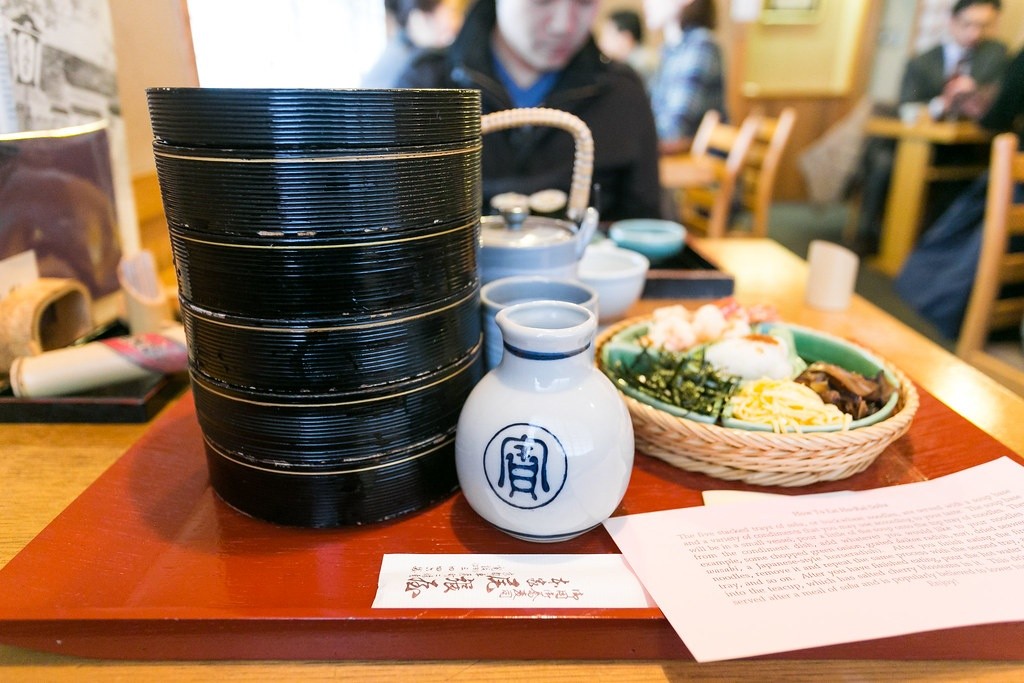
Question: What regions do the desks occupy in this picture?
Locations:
[862,115,998,274]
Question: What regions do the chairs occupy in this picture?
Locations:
[679,109,763,238]
[952,132,1024,394]
[724,107,807,235]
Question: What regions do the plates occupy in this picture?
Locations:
[599,319,902,433]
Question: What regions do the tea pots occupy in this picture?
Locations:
[480,108,598,286]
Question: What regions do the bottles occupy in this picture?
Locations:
[455,303,635,544]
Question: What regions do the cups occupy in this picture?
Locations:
[480,274,599,370]
[805,239,859,311]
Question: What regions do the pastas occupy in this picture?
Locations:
[728,378,853,437]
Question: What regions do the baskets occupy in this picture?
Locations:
[592,305,919,488]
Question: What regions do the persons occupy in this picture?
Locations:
[848,1,1023,259]
[367,0,742,227]
[394,0,659,220]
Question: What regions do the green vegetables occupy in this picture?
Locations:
[608,341,736,418]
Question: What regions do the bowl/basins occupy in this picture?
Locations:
[575,244,647,317]
[608,216,685,261]
